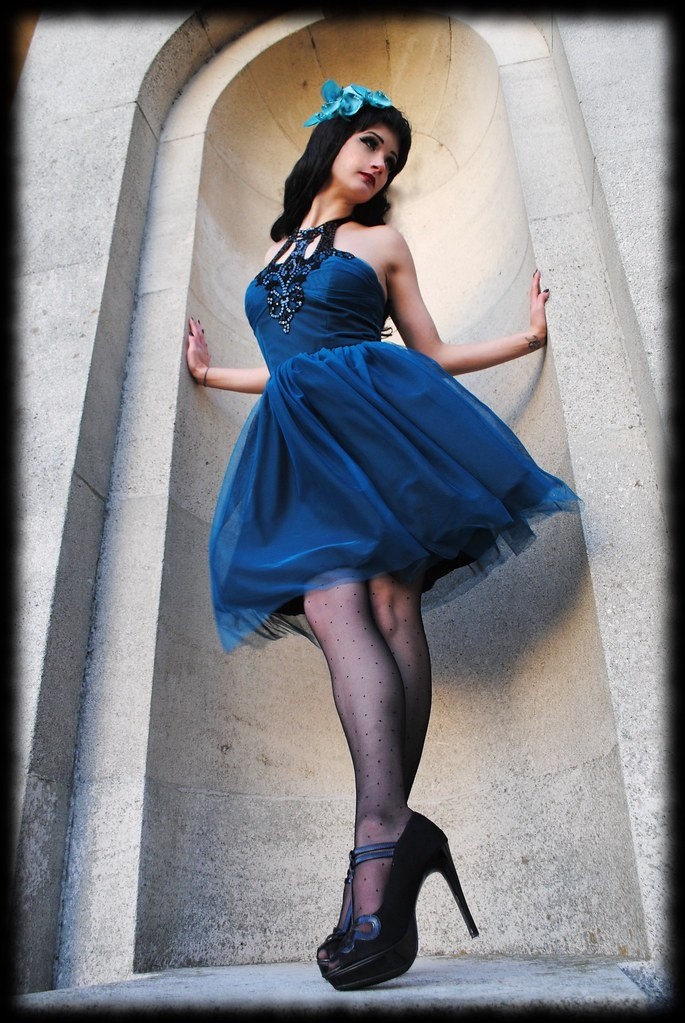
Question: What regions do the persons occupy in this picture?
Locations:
[185,78,553,993]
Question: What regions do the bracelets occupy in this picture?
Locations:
[203,366,210,388]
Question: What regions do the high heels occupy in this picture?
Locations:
[316,851,353,979]
[326,812,479,992]
